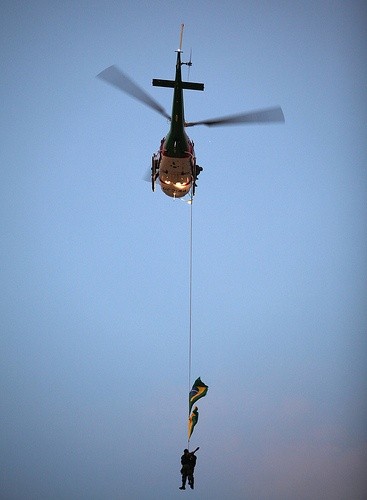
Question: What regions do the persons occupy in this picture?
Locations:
[178,449,197,490]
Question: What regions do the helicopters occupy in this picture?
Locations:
[97,20,285,199]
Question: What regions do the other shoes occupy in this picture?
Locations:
[179,486,186,490]
[188,482,193,489]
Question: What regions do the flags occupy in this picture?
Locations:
[189,377,208,414]
[188,407,200,441]
